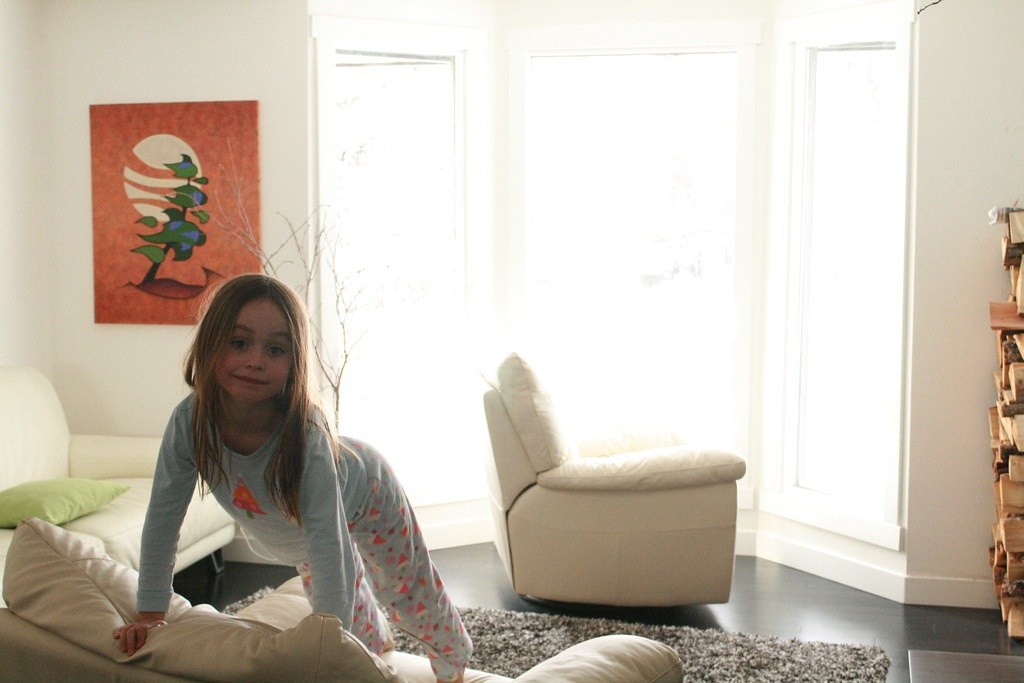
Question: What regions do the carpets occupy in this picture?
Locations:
[221,585,893,683]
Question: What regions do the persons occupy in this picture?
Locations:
[112,274,473,683]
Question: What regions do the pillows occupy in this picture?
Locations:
[0,476,131,528]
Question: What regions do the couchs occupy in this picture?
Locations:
[0,607,685,683]
[0,361,236,608]
[482,353,746,611]
